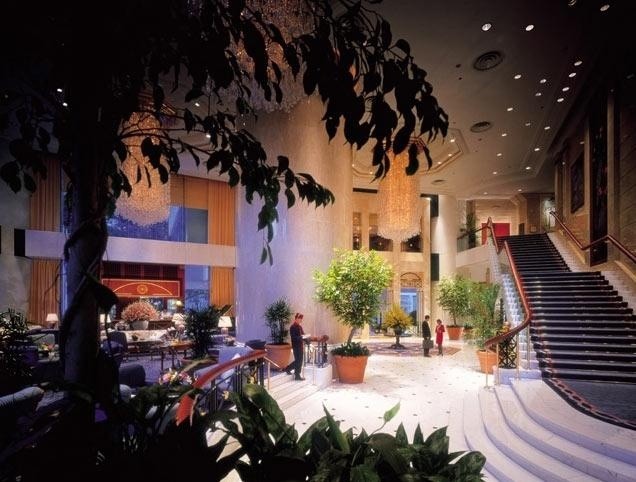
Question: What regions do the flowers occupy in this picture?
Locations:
[120,300,160,322]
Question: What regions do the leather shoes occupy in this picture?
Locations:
[295,377,305,380]
[283,368,292,375]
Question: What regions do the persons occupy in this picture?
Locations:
[421,315,446,356]
[282,314,311,380]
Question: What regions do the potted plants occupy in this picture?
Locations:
[263,295,296,371]
[436,271,504,376]
[175,304,232,379]
[312,245,417,384]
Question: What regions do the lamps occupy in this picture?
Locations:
[45,313,58,328]
[217,316,232,334]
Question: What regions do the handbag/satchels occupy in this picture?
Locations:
[423,339,433,349]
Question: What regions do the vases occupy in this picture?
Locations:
[130,319,149,330]
